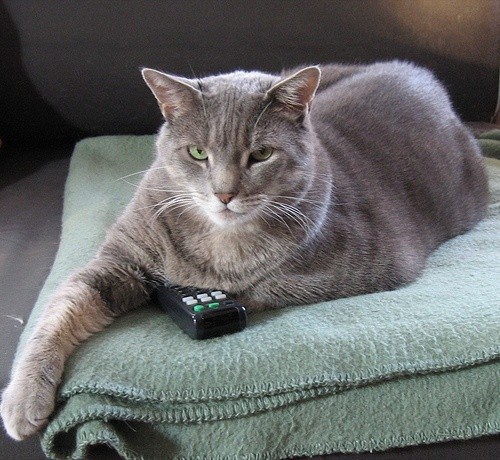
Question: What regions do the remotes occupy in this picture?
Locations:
[155,280,249,341]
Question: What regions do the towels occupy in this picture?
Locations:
[9,130,500,460]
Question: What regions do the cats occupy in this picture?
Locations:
[0,58,491,443]
